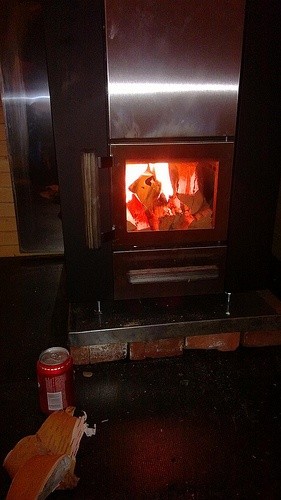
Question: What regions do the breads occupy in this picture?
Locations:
[3,408,86,481]
[6,454,73,500]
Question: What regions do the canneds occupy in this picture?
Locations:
[36,346,75,416]
